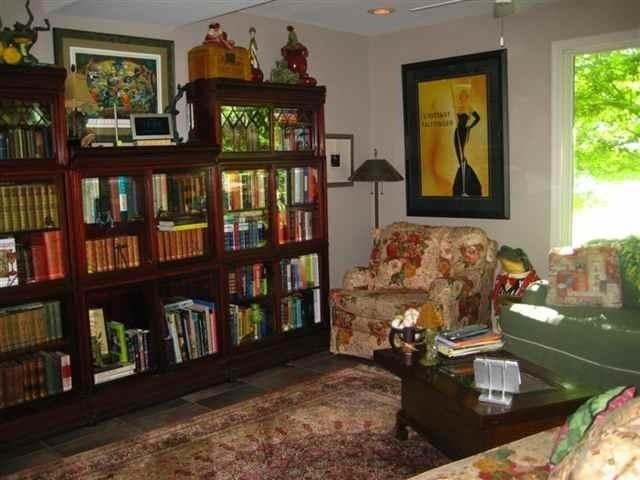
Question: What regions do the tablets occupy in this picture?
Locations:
[130,112,175,141]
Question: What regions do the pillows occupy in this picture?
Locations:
[544,243,625,310]
[547,384,635,464]
[548,398,639,480]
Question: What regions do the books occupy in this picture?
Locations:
[220,164,318,251]
[88,308,154,383]
[436,323,504,358]
[1,126,52,159]
[1,181,60,232]
[85,223,203,274]
[161,294,217,365]
[2,299,72,407]
[1,230,66,285]
[228,252,322,349]
[81,170,206,226]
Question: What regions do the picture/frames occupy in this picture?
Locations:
[52,28,176,142]
[399,48,512,220]
[321,132,355,186]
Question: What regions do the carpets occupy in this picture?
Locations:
[0,363,454,480]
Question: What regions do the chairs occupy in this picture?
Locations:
[328,220,499,368]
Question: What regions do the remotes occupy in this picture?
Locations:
[445,325,488,341]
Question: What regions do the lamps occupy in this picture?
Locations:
[348,159,404,230]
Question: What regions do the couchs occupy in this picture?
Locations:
[400,423,640,480]
[498,235,640,382]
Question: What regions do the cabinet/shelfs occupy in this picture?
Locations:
[0,63,328,449]
